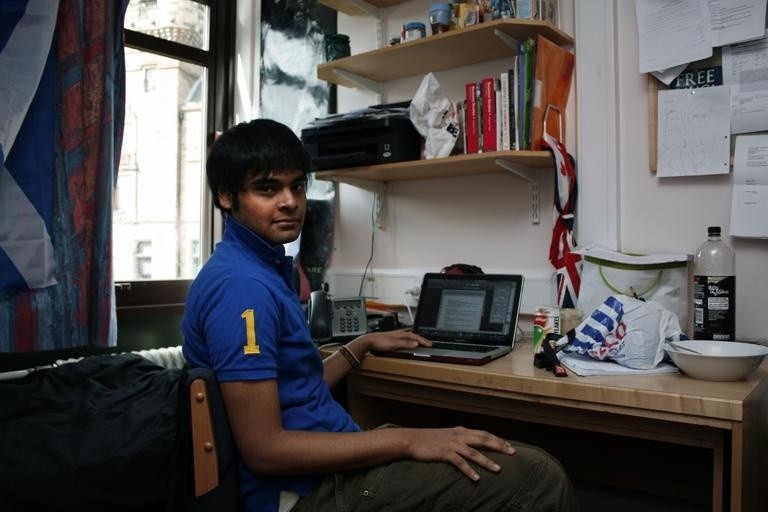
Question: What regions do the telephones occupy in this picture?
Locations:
[307,292,369,343]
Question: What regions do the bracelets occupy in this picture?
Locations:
[336,343,362,372]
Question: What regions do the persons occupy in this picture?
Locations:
[180,117,576,511]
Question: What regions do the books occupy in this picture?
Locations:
[455,34,574,155]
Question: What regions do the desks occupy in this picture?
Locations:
[308,305,765,512]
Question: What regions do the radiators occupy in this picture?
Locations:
[0,342,191,378]
[170,371,258,512]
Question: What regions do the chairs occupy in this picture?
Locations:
[200,417,206,420]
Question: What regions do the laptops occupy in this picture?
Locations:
[371,271,523,366]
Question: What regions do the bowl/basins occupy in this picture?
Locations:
[661,340,768,381]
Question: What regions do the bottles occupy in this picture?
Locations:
[404,22,426,42]
[693,227,735,342]
[429,3,450,35]
[533,306,560,355]
